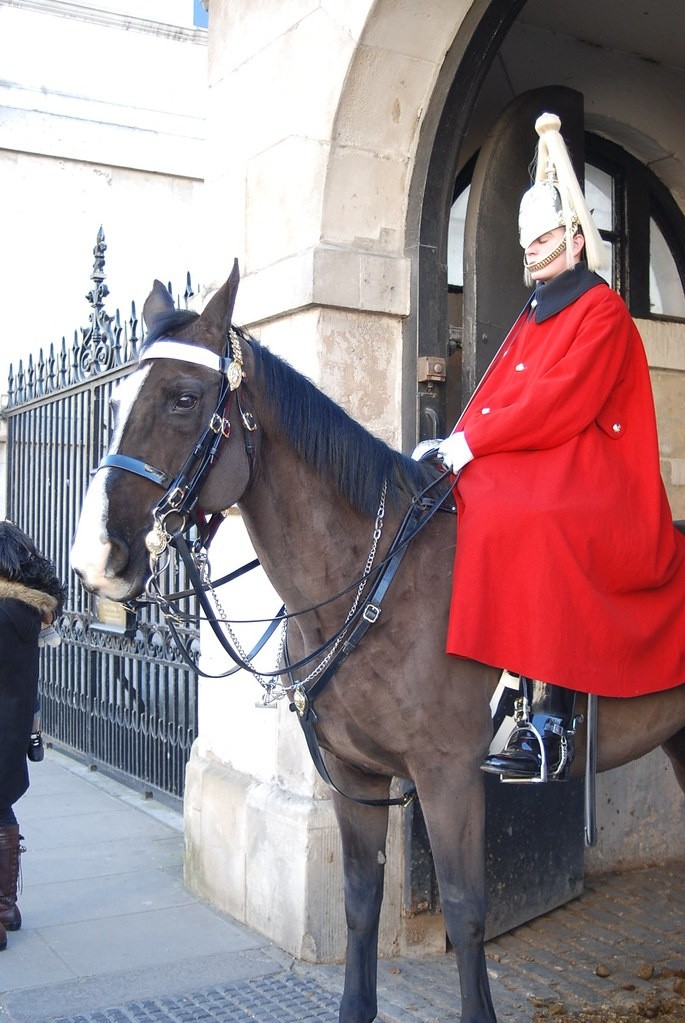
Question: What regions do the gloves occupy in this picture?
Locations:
[436,431,474,476]
[38,620,61,647]
[411,439,443,461]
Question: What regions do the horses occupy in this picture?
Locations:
[64,257,685,1023]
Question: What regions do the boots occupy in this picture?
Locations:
[0,824,22,951]
[479,679,576,782]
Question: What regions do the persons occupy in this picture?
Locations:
[0,520,67,951]
[411,112,685,777]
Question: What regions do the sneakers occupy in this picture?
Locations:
[27,731,44,761]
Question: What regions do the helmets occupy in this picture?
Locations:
[518,112,605,287]
